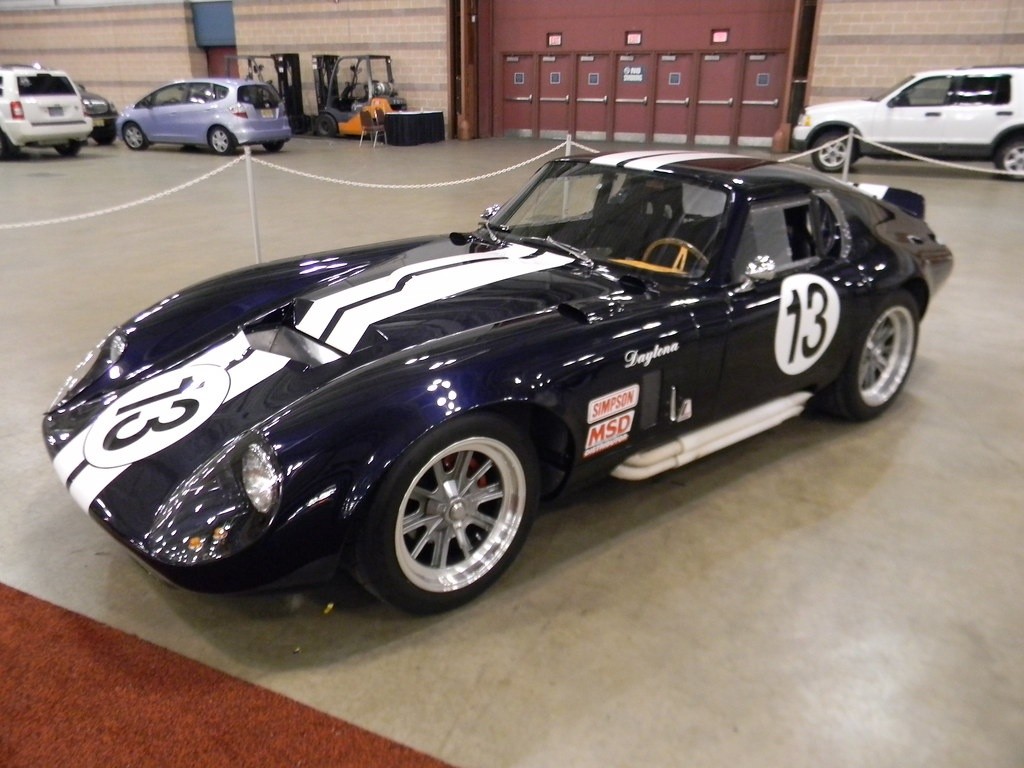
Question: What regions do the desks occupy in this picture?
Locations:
[385,111,445,146]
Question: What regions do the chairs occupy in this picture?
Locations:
[358,110,387,149]
[549,181,649,253]
[374,107,385,126]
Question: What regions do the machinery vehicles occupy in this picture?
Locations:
[313,53,407,138]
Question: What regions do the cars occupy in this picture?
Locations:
[73,79,120,147]
[116,77,294,154]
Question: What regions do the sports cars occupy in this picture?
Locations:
[42,147,954,616]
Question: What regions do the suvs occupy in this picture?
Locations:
[0,61,95,163]
[791,64,1024,182]
